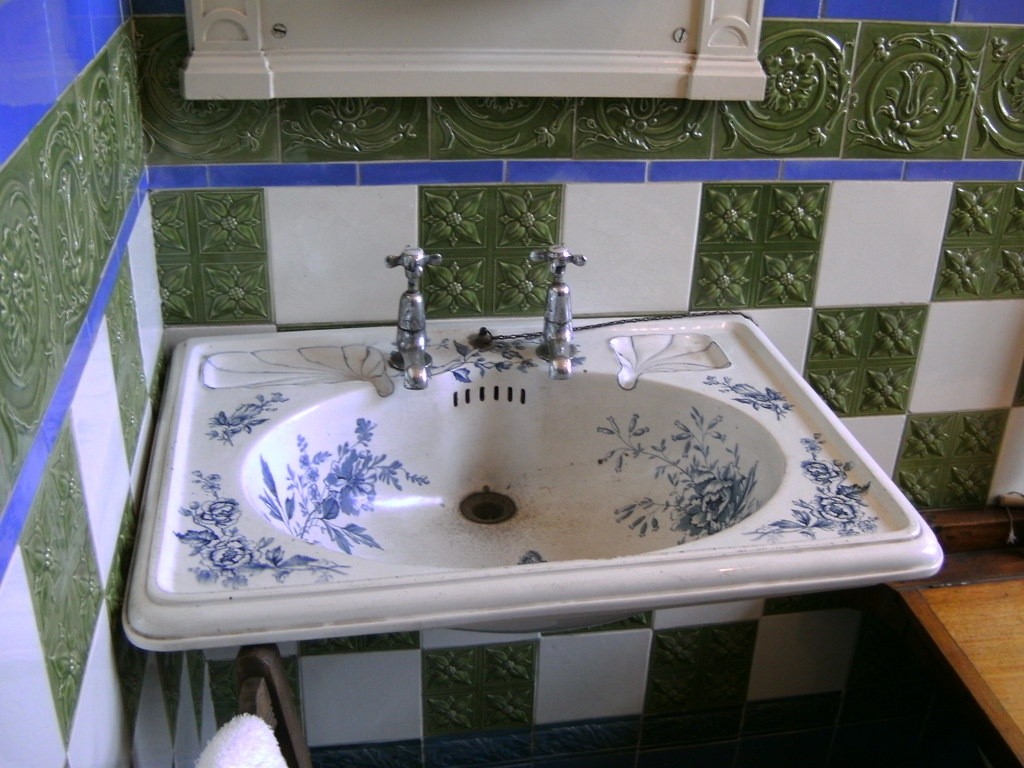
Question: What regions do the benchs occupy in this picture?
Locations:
[892,507,1024,768]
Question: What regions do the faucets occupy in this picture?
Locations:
[385,245,443,390]
[530,245,587,379]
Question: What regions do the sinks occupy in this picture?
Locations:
[120,313,945,652]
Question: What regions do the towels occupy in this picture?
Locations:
[194,712,289,768]
